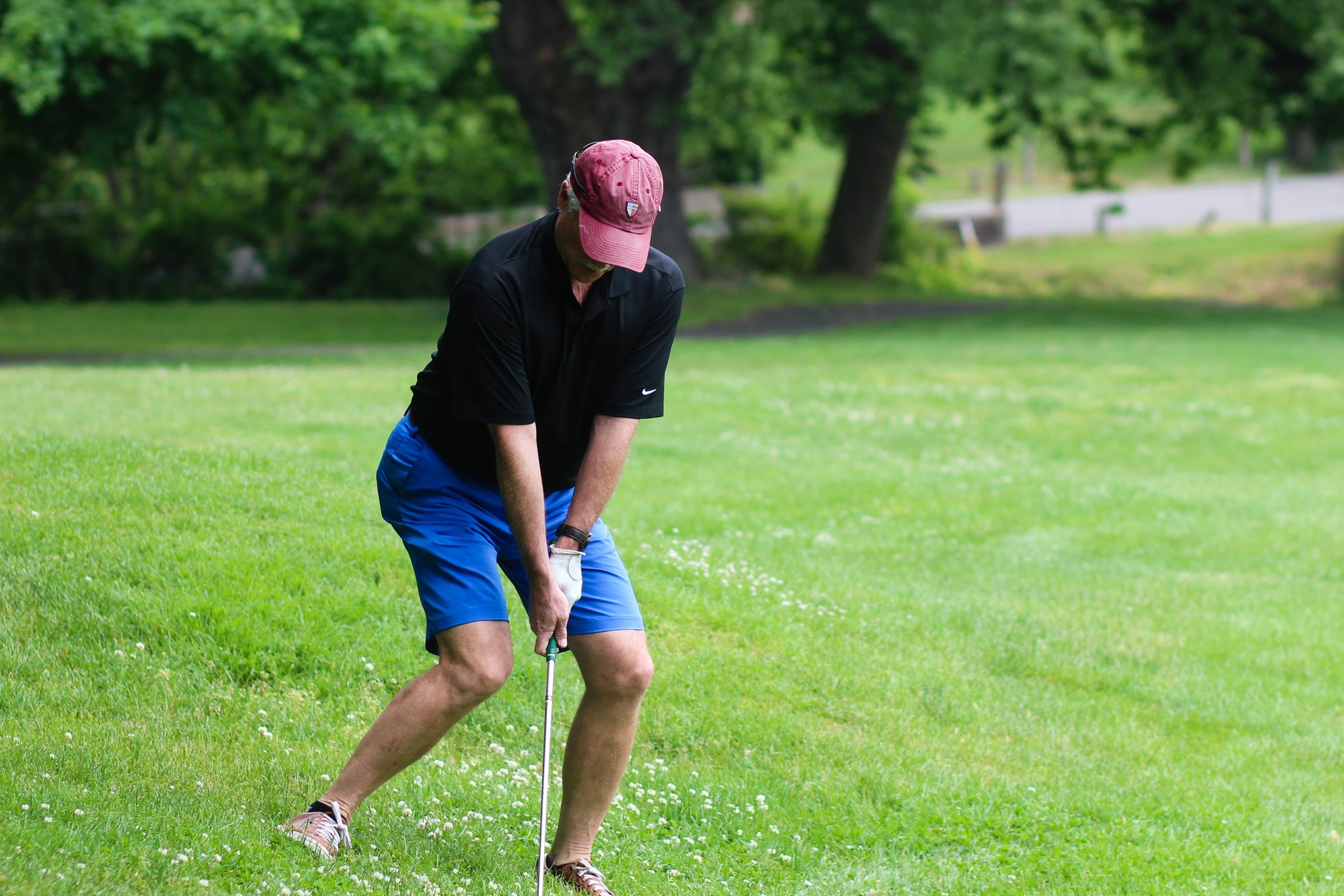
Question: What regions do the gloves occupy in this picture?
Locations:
[548,545,585,611]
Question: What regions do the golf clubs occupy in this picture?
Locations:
[537,637,558,896]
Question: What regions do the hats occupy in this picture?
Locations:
[570,140,664,271]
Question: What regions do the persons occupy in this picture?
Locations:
[277,139,685,896]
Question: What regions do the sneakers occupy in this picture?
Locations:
[276,800,353,863]
[537,853,614,896]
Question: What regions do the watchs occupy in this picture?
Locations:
[555,524,593,552]
